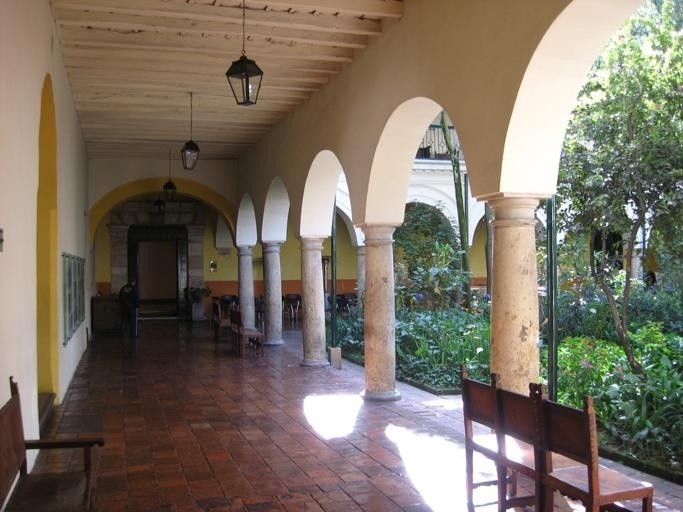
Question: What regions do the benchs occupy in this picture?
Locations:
[0,376,106,512]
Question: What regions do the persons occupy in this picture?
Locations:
[117,276,139,336]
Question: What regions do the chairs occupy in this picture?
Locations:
[495,373,586,512]
[460,362,524,512]
[537,379,655,512]
[207,283,357,364]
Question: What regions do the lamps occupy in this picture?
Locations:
[225,0,266,107]
[181,90,201,171]
[160,144,177,199]
